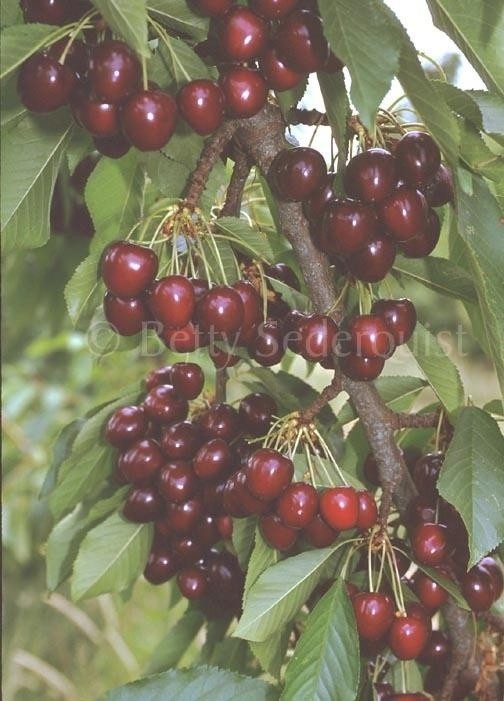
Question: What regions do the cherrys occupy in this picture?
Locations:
[16,0,503,700]
